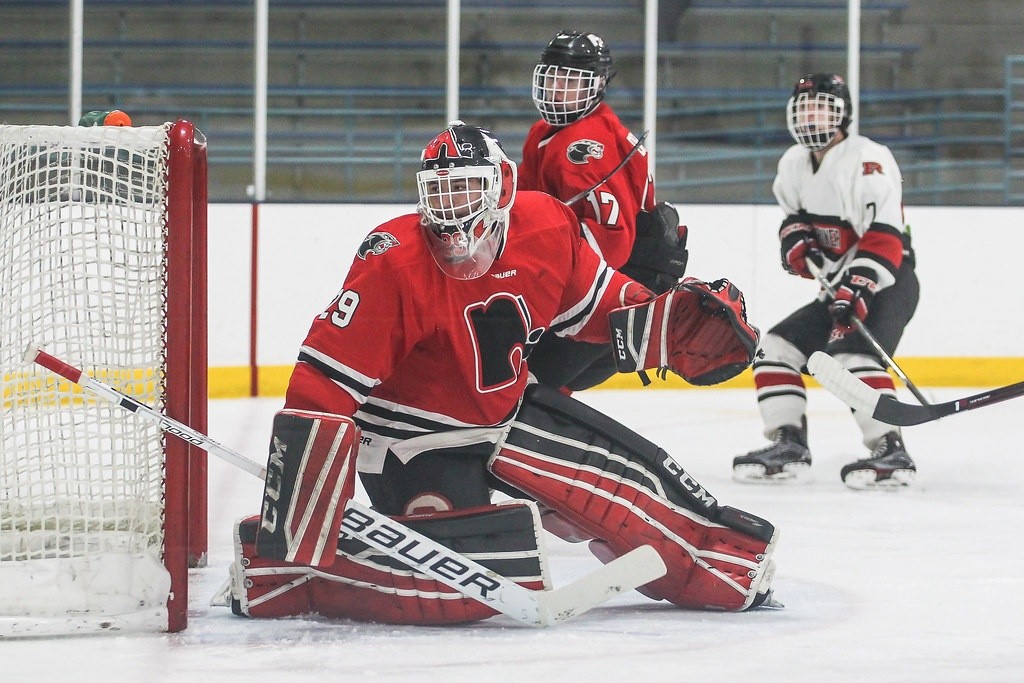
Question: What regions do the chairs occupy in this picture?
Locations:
[0,0,919,203]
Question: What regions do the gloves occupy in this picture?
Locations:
[778,215,823,280]
[829,270,879,333]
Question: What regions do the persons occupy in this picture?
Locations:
[733,73,917,489]
[511,29,689,394]
[233,122,785,627]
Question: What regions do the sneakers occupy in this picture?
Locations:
[841,431,916,490]
[733,415,811,483]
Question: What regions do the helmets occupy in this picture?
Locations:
[532,29,612,125]
[786,72,851,151]
[415,120,518,262]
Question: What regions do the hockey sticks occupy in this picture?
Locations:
[21,347,672,630]
[805,255,931,404]
[805,351,1023,424]
[566,130,649,204]
